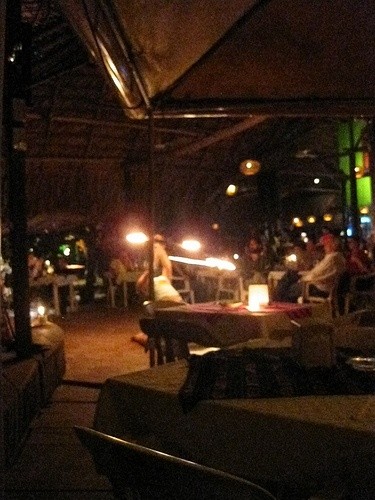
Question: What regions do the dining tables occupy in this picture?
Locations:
[154,300,311,362]
[36,275,75,317]
[93,328,375,500]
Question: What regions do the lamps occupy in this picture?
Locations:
[239,158,261,176]
[248,284,269,305]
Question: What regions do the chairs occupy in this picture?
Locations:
[139,320,235,368]
[104,268,373,327]
[76,424,275,499]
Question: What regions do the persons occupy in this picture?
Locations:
[138,243,190,361]
[27,251,49,280]
[239,231,374,304]
[83,250,134,295]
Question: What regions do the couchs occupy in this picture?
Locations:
[0,318,66,482]
